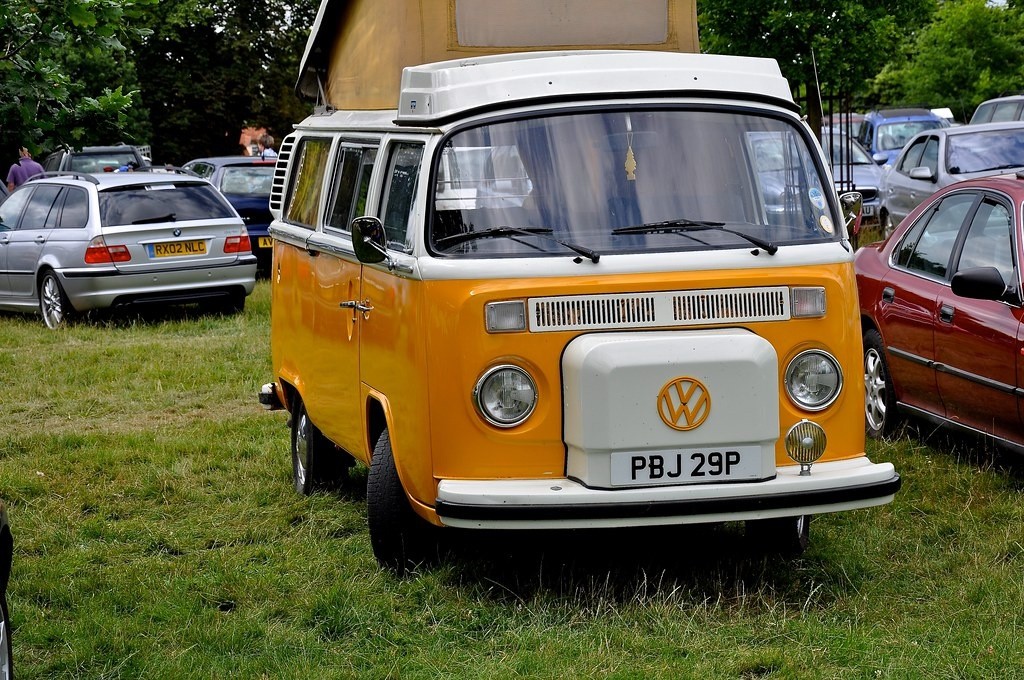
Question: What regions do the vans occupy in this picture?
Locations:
[253,48,898,577]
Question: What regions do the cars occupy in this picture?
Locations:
[0,165,258,331]
[35,141,280,281]
[850,171,1024,464]
[742,93,1024,255]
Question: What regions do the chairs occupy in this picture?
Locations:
[882,134,895,149]
[606,191,746,230]
[434,208,530,250]
[225,177,249,192]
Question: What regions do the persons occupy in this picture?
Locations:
[5,134,278,193]
